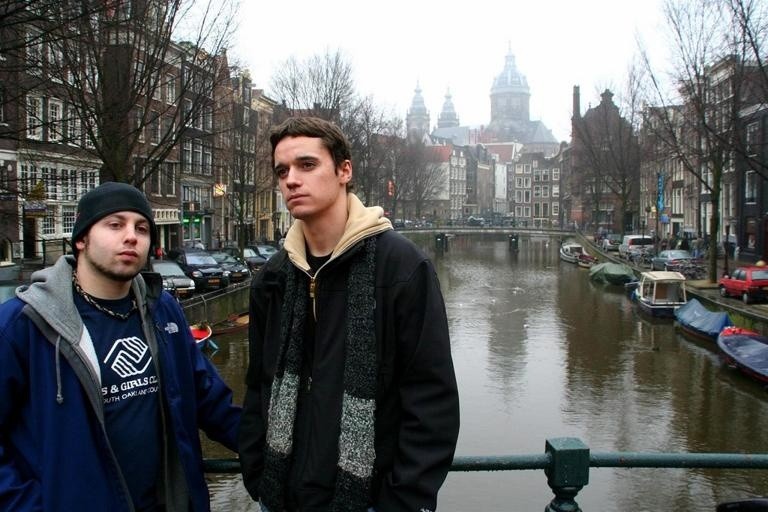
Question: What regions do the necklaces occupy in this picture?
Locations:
[71,267,139,321]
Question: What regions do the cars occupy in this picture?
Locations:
[593,227,623,251]
[145,236,285,301]
[394,217,434,231]
[650,248,700,274]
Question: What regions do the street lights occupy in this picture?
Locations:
[640,212,646,247]
[606,208,611,234]
[274,209,281,242]
[722,216,732,277]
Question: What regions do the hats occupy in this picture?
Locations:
[69,180,161,262]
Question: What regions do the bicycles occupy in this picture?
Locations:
[675,257,711,281]
[633,248,653,267]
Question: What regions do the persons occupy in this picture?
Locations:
[240,117,460,512]
[421,215,426,230]
[195,239,204,251]
[215,230,223,249]
[651,230,689,255]
[273,223,288,249]
[1,183,242,511]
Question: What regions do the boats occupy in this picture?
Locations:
[717,324,767,383]
[557,240,588,264]
[212,309,248,335]
[587,262,634,284]
[623,280,640,292]
[674,296,731,342]
[632,270,688,319]
[576,254,598,269]
[188,321,216,355]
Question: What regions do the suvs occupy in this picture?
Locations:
[617,234,658,262]
[718,261,767,305]
